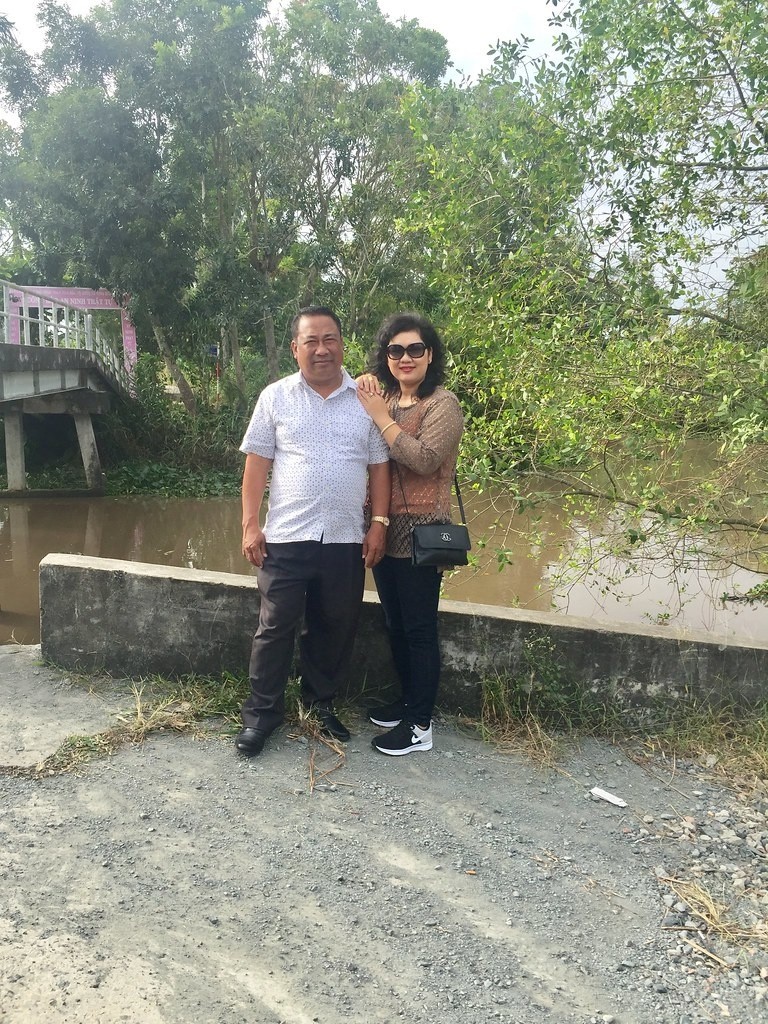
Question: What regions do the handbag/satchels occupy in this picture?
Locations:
[409,523,471,568]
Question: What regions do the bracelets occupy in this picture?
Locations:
[381,421,396,435]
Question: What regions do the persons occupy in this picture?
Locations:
[355,312,464,756]
[236,305,391,752]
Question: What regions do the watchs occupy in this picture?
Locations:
[370,516,389,526]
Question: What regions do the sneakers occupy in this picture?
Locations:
[368,700,408,727]
[371,719,434,756]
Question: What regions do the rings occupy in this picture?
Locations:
[379,557,381,559]
[369,393,373,398]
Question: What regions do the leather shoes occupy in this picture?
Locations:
[236,726,263,756]
[312,708,351,742]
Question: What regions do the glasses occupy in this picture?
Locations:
[385,342,428,361]
